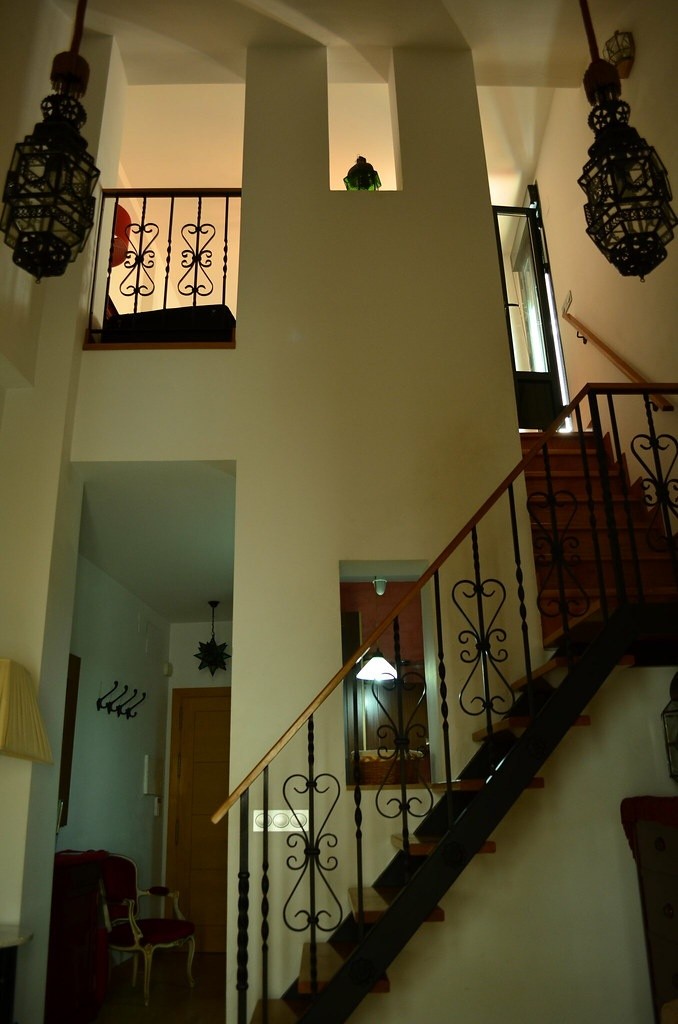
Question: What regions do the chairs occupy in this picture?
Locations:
[93,853,196,1007]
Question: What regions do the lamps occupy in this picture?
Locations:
[603,29,634,79]
[356,576,397,681]
[555,0,678,281]
[0,0,101,283]
[193,601,231,676]
